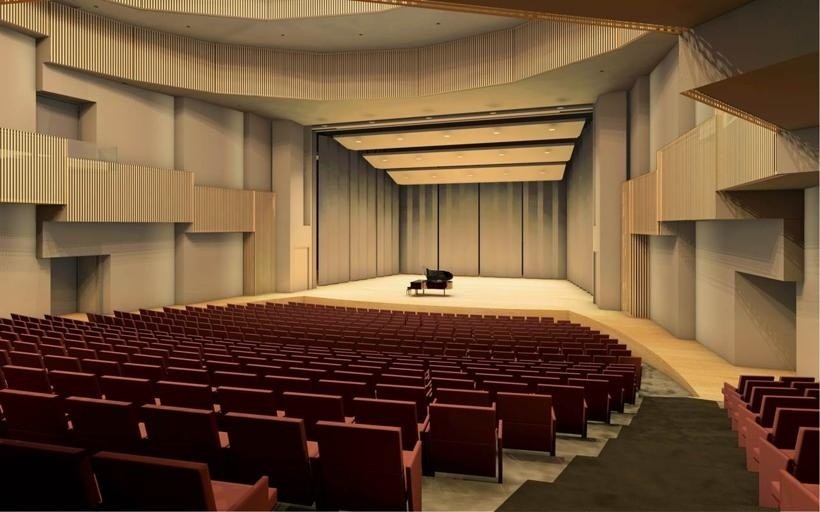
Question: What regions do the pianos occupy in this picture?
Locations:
[407,268,453,295]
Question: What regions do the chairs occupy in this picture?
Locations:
[720,372,819,511]
